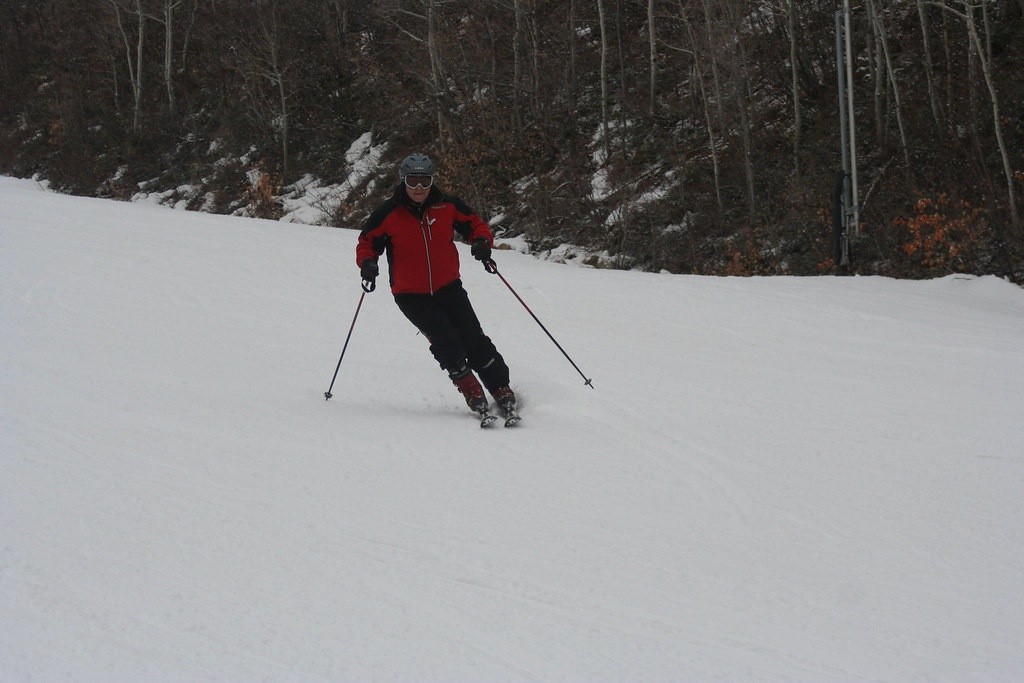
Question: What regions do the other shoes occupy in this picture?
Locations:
[461,382,488,410]
[490,383,516,407]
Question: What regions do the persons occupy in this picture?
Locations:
[356,154,521,427]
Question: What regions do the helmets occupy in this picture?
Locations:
[399,152,435,183]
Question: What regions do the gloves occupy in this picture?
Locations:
[470,237,492,261]
[360,261,380,281]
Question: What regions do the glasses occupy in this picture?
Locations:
[404,174,433,189]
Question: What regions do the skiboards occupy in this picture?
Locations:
[467,388,523,429]
